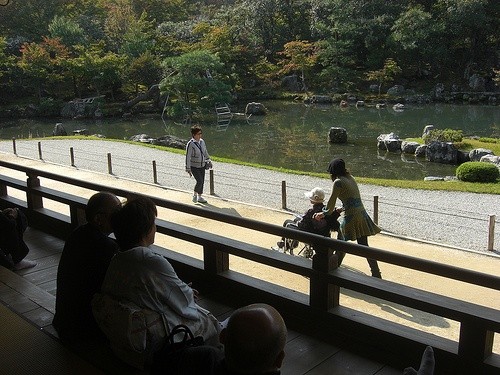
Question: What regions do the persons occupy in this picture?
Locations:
[169,303,289,375]
[185,127,214,203]
[277,187,334,250]
[99,198,232,370]
[404,346,435,375]
[52,194,122,351]
[0,207,37,271]
[312,158,381,279]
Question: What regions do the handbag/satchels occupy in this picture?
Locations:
[148,324,211,375]
[204,159,212,170]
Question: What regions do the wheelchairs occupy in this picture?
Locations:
[272,206,336,262]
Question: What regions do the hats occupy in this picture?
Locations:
[304,187,325,203]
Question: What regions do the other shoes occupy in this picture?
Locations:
[193,195,198,203]
[277,242,289,251]
[198,196,207,203]
